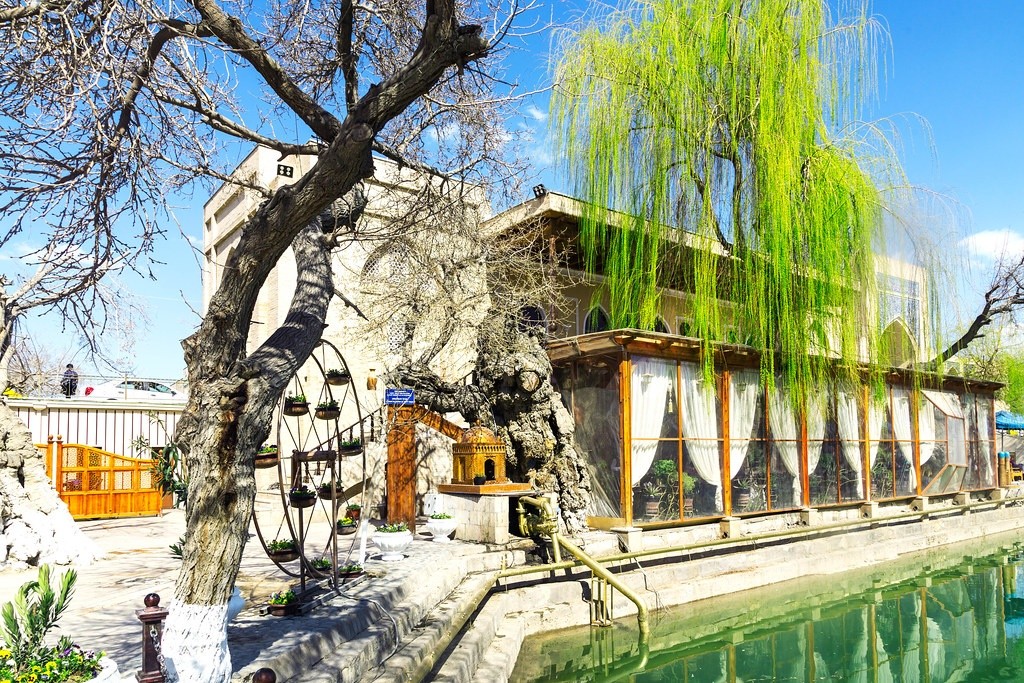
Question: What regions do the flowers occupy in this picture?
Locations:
[328,368,349,378]
[337,516,358,526]
[284,393,307,403]
[317,397,338,408]
[267,589,299,605]
[290,484,316,496]
[267,537,299,551]
[341,436,361,446]
[310,556,333,568]
[255,442,278,453]
[429,511,453,519]
[375,522,408,534]
[338,561,363,571]
[319,479,341,488]
[0,637,120,683]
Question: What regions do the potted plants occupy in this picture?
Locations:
[647,458,678,488]
[737,476,752,508]
[473,473,487,485]
[344,504,362,521]
[674,471,698,516]
[638,481,666,518]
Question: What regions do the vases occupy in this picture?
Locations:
[338,571,363,580]
[266,548,301,562]
[254,452,280,469]
[341,445,363,456]
[308,567,332,576]
[315,407,341,420]
[427,518,457,542]
[317,486,344,501]
[335,524,358,535]
[326,374,352,386]
[271,605,298,617]
[285,402,311,416]
[5,648,122,683]
[374,531,414,561]
[289,494,316,508]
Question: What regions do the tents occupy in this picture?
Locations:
[996,411,1024,452]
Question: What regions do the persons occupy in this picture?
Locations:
[60,364,79,395]
